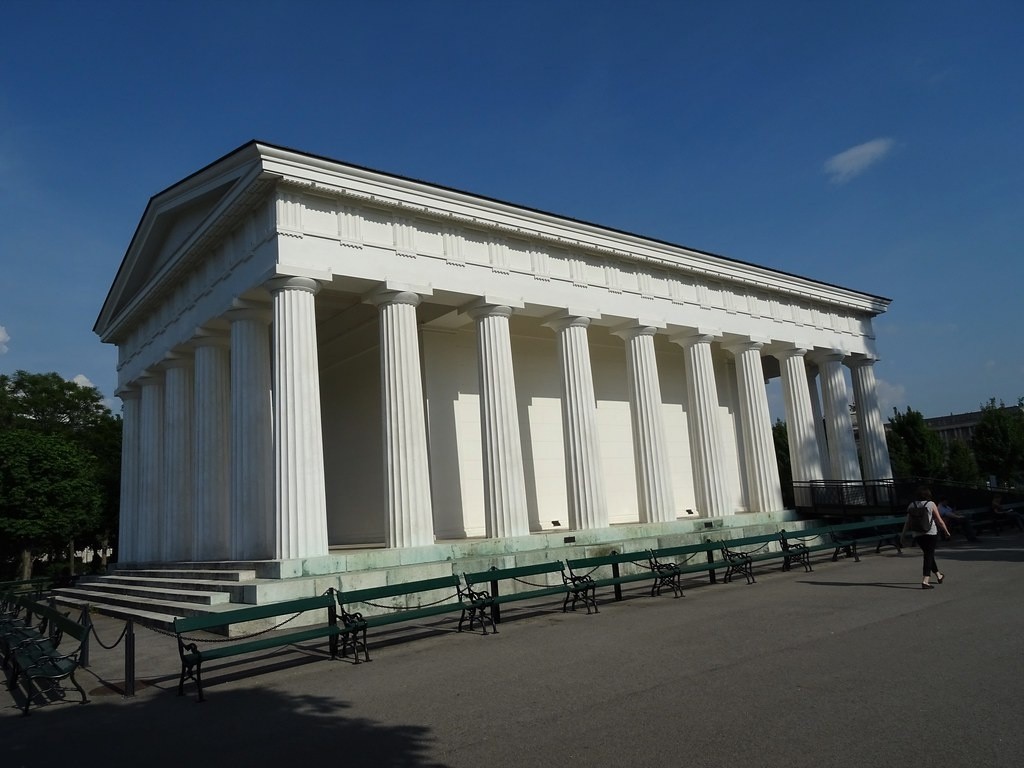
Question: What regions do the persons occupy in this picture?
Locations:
[900,483,952,590]
[934,494,982,543]
[990,492,1023,533]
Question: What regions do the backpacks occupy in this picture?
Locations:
[909,500,933,534]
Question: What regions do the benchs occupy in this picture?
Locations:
[649,539,754,598]
[336,573,489,663]
[172,592,362,705]
[783,502,1023,572]
[720,530,811,584]
[0,587,94,716]
[463,558,594,634]
[566,549,682,614]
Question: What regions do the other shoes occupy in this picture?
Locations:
[938,574,944,584]
[922,582,934,589]
